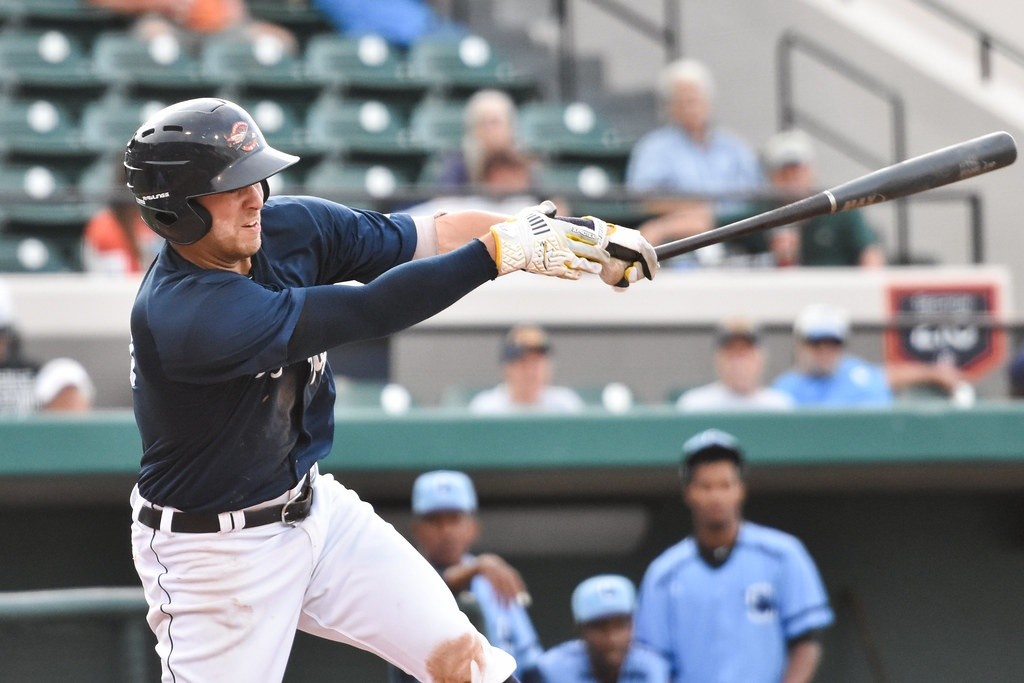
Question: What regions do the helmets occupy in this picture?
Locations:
[125,96,301,246]
[675,431,752,497]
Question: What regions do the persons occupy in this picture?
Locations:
[537,573,675,683]
[636,429,836,683]
[411,469,542,683]
[120,98,660,683]
[0,1,883,417]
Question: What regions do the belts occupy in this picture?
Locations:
[139,475,315,534]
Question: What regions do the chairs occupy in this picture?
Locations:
[1,0,766,272]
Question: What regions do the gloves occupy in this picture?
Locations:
[489,198,611,281]
[583,213,659,293]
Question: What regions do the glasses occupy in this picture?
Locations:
[504,343,550,362]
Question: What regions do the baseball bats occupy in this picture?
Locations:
[598,129,1019,283]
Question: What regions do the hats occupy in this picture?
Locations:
[754,131,811,175]
[791,300,849,345]
[411,471,478,518]
[714,306,759,346]
[568,575,637,629]
